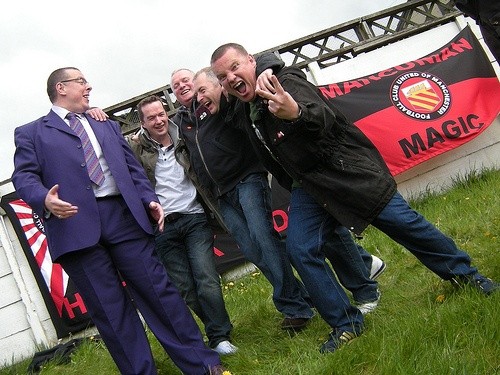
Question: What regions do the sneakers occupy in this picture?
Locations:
[369,253,387,281]
[281,317,312,329]
[462,272,494,297]
[355,288,382,319]
[210,340,239,356]
[319,330,364,354]
[212,365,235,375]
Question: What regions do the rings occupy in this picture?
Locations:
[58,215,61,218]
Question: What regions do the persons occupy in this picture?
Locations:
[86,42,498,355]
[11,67,232,375]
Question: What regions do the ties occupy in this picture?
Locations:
[65,112,106,187]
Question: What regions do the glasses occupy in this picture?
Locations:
[61,76,89,86]
